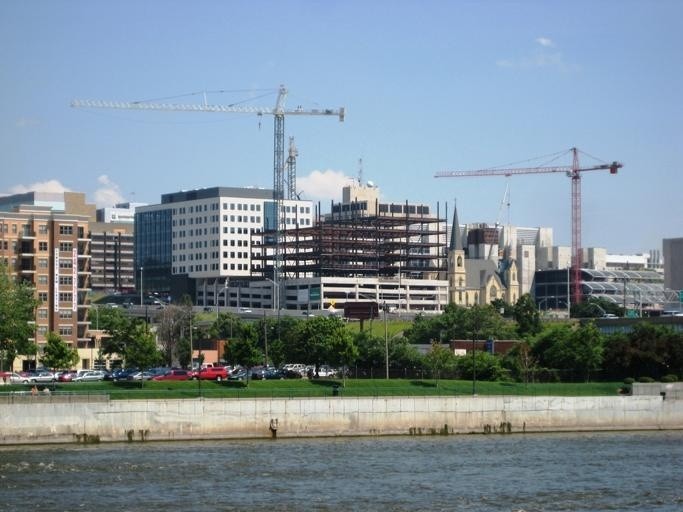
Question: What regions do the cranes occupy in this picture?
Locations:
[429,149,628,317]
[283,132,305,201]
[69,85,354,310]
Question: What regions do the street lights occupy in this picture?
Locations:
[262,277,281,314]
[215,282,231,327]
[138,266,147,308]
[271,266,278,311]
[377,302,392,380]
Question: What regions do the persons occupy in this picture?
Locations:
[42,386,51,396]
[31,385,38,396]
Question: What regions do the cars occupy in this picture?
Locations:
[241,306,254,313]
[123,302,134,309]
[203,306,216,313]
[303,311,315,317]
[0,360,338,384]
[599,301,683,321]
[147,290,164,307]
[107,301,119,310]
